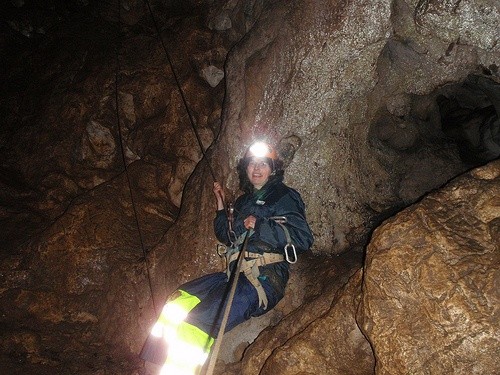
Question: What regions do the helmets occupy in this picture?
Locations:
[244,141,277,160]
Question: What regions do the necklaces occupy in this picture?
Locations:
[140,141,314,375]
